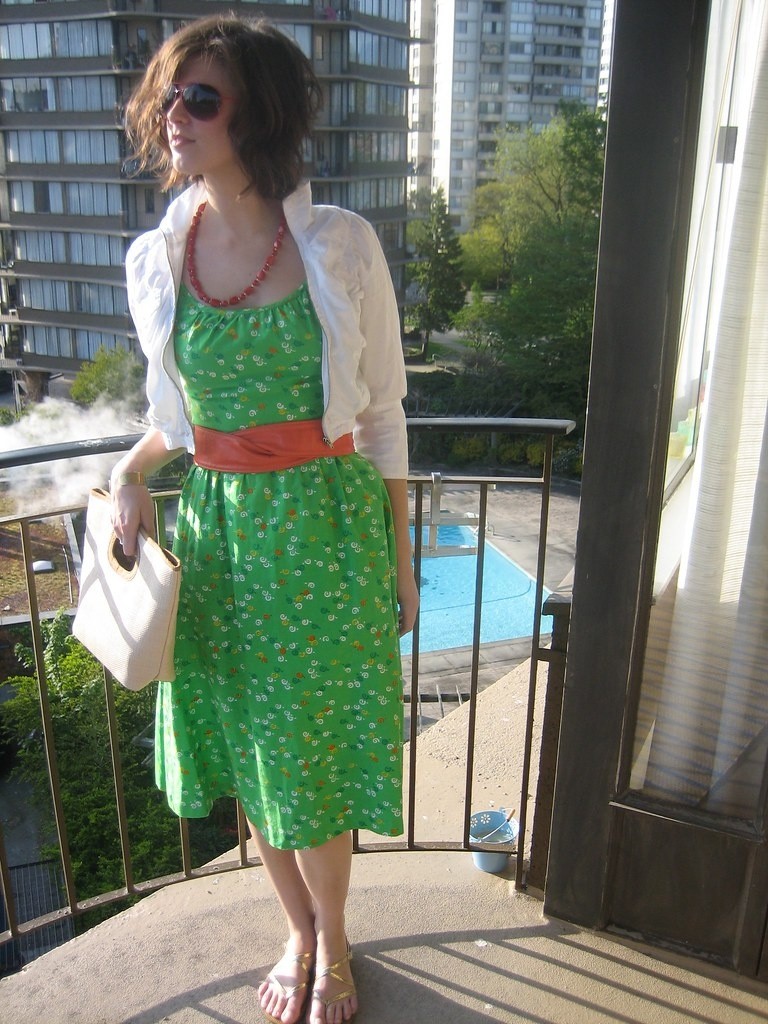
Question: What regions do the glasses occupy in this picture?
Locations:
[160,81,244,122]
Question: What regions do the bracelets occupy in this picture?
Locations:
[111,471,146,488]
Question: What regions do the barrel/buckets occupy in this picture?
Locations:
[467,809,519,873]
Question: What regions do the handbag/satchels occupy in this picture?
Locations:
[72,487,183,692]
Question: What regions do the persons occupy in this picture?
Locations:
[110,18,422,1024]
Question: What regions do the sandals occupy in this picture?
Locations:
[260,943,316,1024]
[304,932,357,1024]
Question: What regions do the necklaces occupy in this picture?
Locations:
[186,203,286,308]
